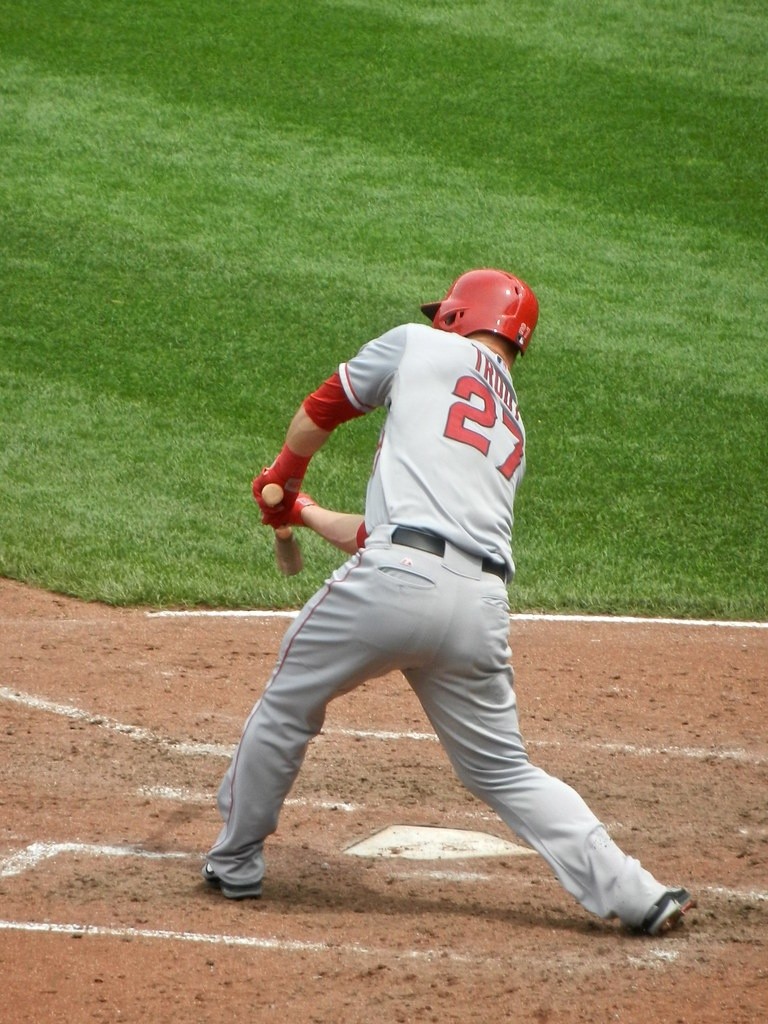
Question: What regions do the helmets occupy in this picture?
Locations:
[420,268,539,357]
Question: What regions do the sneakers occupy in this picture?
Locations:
[643,886,698,937]
[201,862,263,899]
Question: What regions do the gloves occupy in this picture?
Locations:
[287,491,317,527]
[253,444,313,528]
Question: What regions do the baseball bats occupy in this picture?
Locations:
[261,482,305,578]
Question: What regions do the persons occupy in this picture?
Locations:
[203,269,696,934]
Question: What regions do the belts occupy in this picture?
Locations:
[392,525,506,582]
